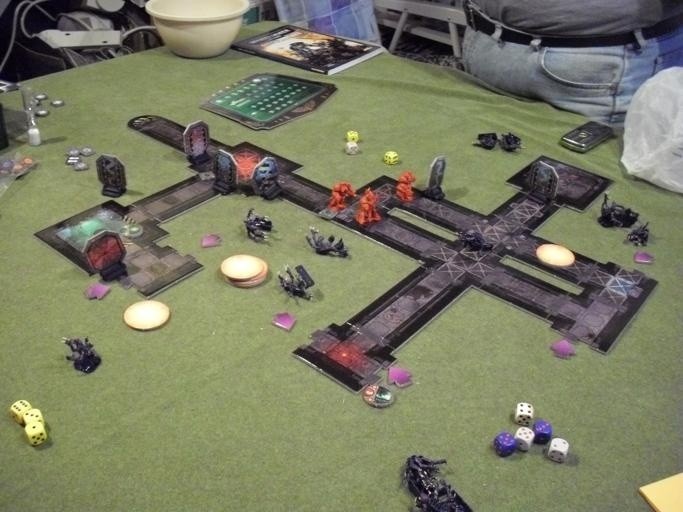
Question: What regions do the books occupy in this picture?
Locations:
[232,23,384,77]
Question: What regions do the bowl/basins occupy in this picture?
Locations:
[144,0,251,59]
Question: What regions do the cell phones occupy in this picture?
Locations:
[560,120,612,153]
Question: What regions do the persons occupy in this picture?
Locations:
[462,0,683,129]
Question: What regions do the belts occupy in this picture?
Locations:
[462,0,683,48]
